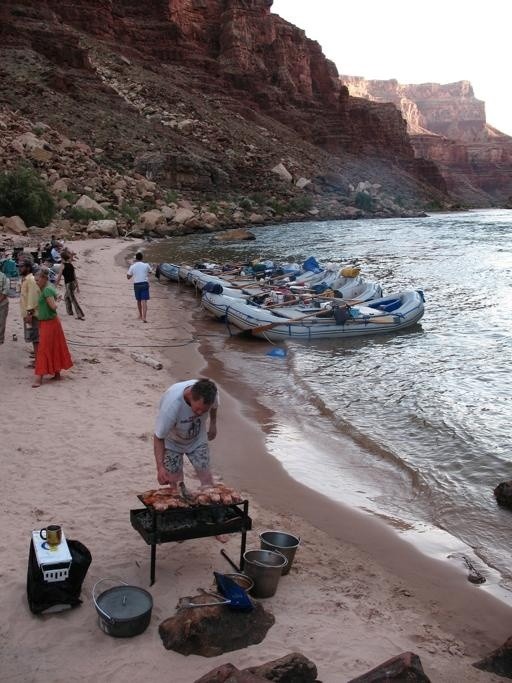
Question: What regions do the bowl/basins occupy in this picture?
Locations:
[214,573,254,594]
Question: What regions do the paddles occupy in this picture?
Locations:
[272,316,400,324]
[252,302,360,335]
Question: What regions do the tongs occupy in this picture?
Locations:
[178,587,230,608]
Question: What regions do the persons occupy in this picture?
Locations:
[1,232,81,316]
[1,271,10,343]
[32,266,73,386]
[127,251,153,322]
[16,259,41,367]
[152,376,230,544]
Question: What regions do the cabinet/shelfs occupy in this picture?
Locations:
[125,489,253,586]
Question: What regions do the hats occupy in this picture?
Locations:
[16,258,33,268]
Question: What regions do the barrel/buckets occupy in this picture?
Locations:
[242,548,288,600]
[259,530,302,577]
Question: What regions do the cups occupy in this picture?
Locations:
[39,524,62,545]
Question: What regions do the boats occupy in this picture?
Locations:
[156,253,431,343]
